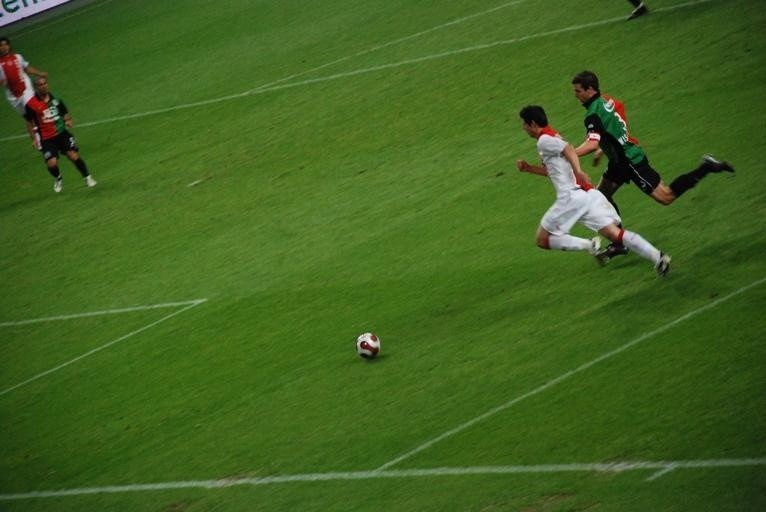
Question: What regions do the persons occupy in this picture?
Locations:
[22,77,97,193]
[516,107,672,277]
[626,0,648,23]
[0,37,48,149]
[567,71,734,260]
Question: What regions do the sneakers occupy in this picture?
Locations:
[625,4,646,19]
[84,175,96,188]
[595,241,628,261]
[699,153,735,173]
[653,253,672,277]
[53,176,61,193]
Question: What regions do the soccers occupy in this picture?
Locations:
[356,333,381,358]
